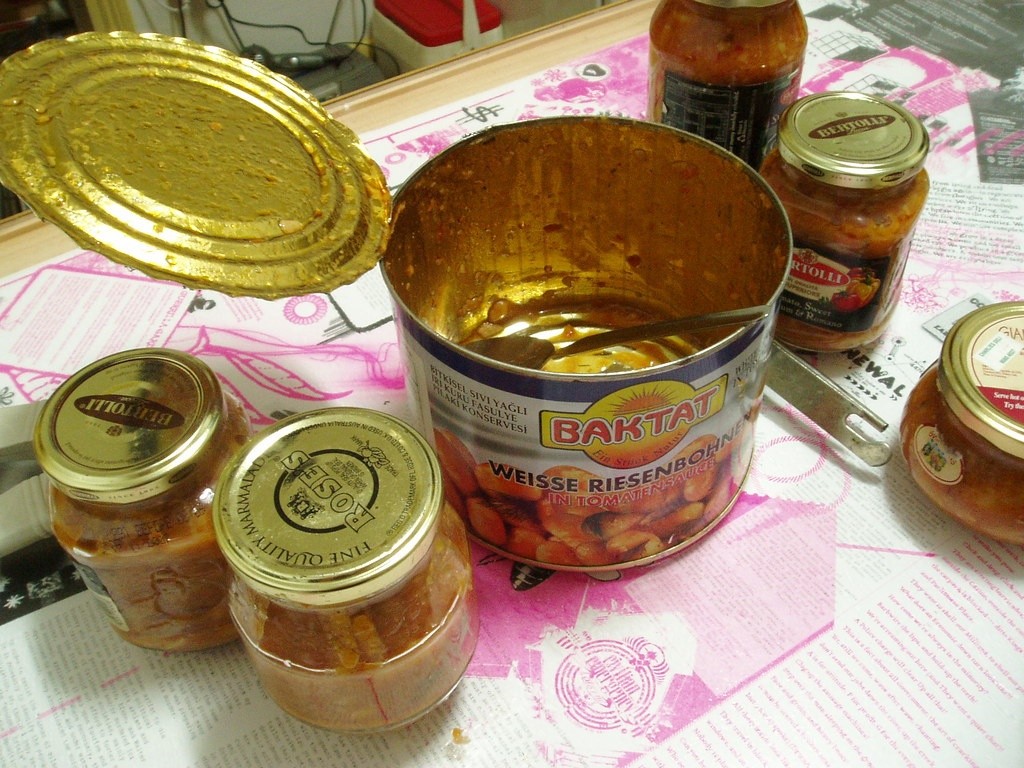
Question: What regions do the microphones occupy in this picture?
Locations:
[240,44,325,71]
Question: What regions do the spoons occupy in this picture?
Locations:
[464,304,773,370]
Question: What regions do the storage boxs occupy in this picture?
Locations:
[369,0,504,80]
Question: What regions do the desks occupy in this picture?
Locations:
[1,0,1023,768]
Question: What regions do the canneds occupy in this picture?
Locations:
[650,0,809,165]
[756,93,932,354]
[33,352,260,654]
[901,301,1024,544]
[211,408,479,735]
